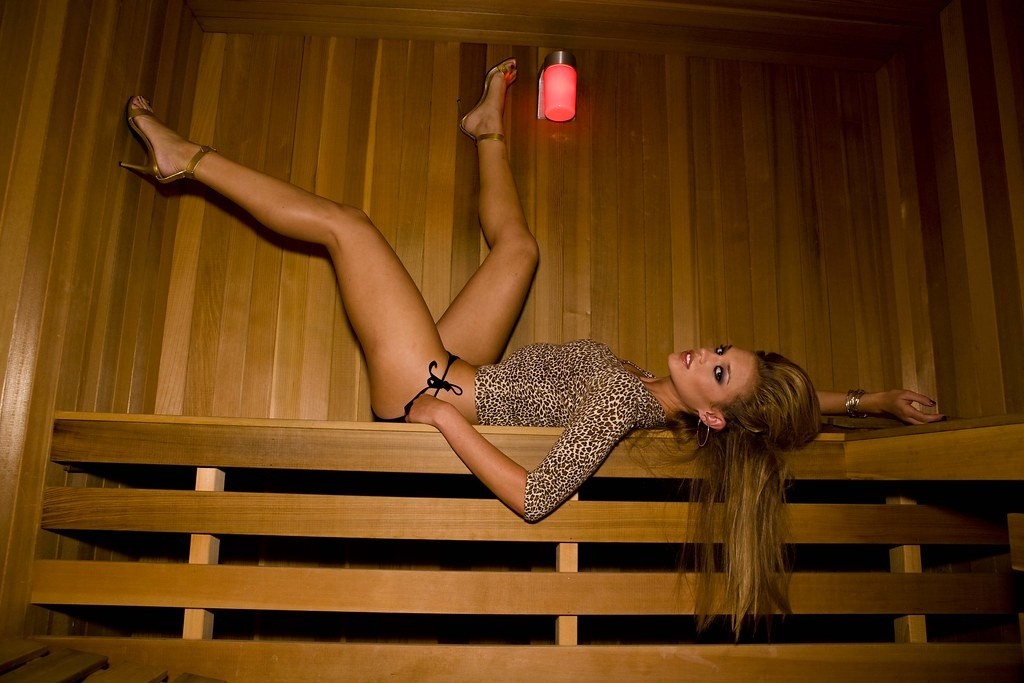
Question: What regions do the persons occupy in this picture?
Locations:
[119,57,949,521]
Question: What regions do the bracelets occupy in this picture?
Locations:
[845,389,864,416]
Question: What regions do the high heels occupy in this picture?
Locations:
[456,56,517,146]
[118,94,216,184]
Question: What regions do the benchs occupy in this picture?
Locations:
[33,410,1024,683]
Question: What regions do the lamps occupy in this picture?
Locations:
[537,51,579,122]
